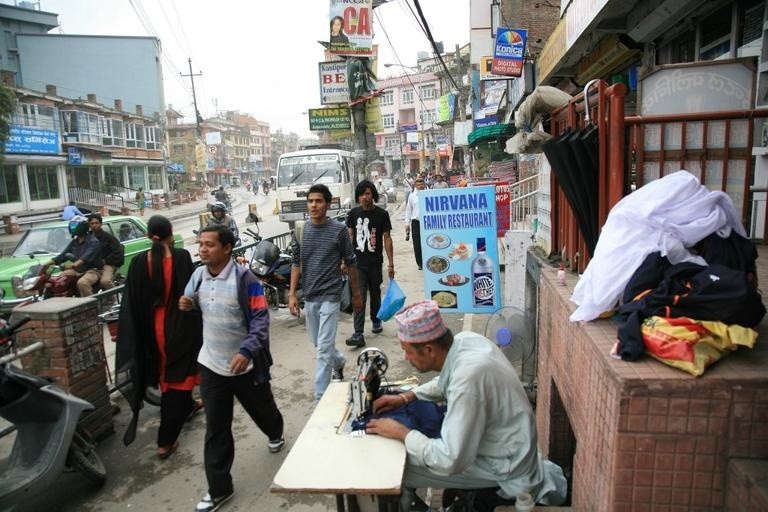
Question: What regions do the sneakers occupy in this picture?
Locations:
[372,323,383,333]
[195,486,236,512]
[346,333,365,347]
[332,366,344,382]
[267,435,286,453]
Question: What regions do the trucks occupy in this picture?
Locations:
[229,176,240,186]
[276,150,360,229]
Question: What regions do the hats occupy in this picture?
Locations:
[393,299,448,344]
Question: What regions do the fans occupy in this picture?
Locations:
[484,306,537,368]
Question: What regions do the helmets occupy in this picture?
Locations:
[209,202,227,213]
[68,215,89,237]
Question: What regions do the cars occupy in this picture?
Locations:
[373,178,397,201]
[0,216,185,296]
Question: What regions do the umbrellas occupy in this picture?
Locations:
[543,79,603,260]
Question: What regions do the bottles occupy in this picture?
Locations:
[471,237,494,308]
[558,263,566,287]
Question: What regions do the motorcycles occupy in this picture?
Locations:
[194,230,245,266]
[214,194,233,210]
[262,183,269,195]
[245,181,251,191]
[253,183,259,194]
[375,188,391,208]
[0,316,108,505]
[240,213,304,310]
[16,251,123,305]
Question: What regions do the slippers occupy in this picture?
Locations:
[185,396,203,419]
[159,438,181,460]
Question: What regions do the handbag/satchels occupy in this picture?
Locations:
[376,277,407,322]
[341,278,355,316]
[169,302,203,375]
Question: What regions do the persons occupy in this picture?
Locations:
[366,299,538,510]
[136,186,149,216]
[400,169,454,271]
[244,177,274,197]
[202,187,238,244]
[330,15,349,49]
[111,216,204,461]
[34,200,135,306]
[341,181,393,346]
[288,184,363,403]
[372,175,388,208]
[179,225,283,512]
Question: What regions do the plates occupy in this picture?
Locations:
[427,255,449,274]
[431,291,458,308]
[426,233,450,249]
[439,277,472,285]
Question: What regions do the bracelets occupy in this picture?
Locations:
[387,264,394,268]
[399,393,410,405]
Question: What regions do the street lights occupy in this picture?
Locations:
[384,62,428,174]
